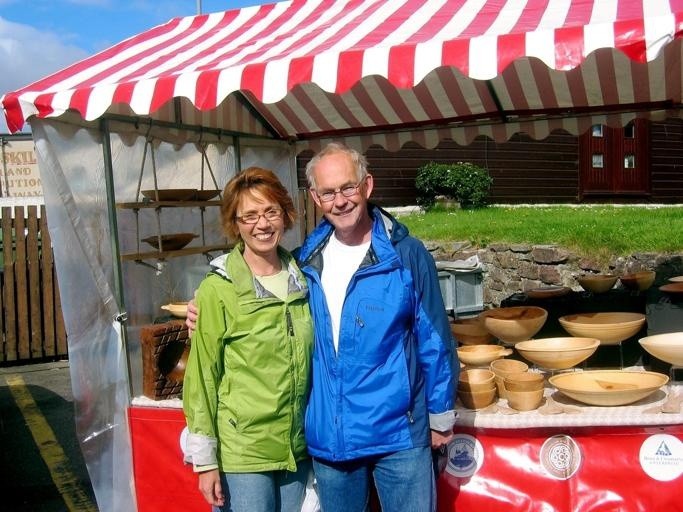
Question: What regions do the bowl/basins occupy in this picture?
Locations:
[142,233,199,253]
[549,368,670,406]
[160,301,188,320]
[660,284,683,308]
[574,274,618,293]
[514,338,602,369]
[455,343,513,366]
[620,272,657,291]
[560,310,646,348]
[446,318,490,346]
[479,304,548,348]
[458,359,544,415]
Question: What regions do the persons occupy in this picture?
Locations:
[175,164,311,512]
[184,141,457,511]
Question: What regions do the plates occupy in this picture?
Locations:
[639,332,683,367]
[137,188,222,201]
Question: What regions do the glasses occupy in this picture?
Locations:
[231,209,284,223]
[316,174,366,202]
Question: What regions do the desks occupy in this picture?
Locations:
[127,357,683,512]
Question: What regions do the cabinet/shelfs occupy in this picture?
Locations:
[114,138,231,261]
